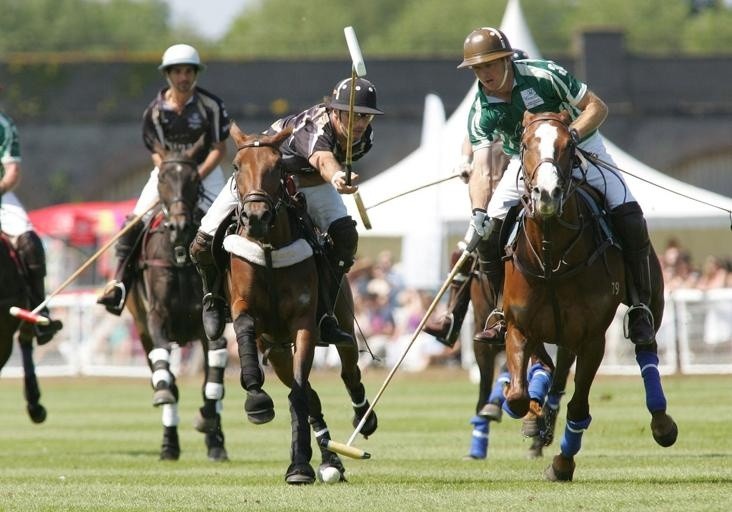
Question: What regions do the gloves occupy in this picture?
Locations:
[464,208,496,248]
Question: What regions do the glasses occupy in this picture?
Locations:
[343,112,375,125]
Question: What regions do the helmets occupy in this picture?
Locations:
[330,78,386,115]
[158,43,207,73]
[456,27,517,69]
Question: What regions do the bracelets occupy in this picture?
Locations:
[331,171,343,190]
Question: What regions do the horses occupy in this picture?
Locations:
[0,160,47,424]
[222,121,378,485]
[467,266,554,462]
[124,131,229,461]
[502,102,679,482]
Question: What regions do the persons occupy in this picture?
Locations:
[420,27,657,347]
[190,75,385,346]
[455,132,512,194]
[95,43,233,317]
[0,237,731,375]
[0,108,63,347]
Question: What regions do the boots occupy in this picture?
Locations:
[474,256,506,345]
[316,260,357,348]
[624,240,656,347]
[422,279,471,348]
[98,244,140,316]
[198,262,225,341]
[28,266,62,346]
